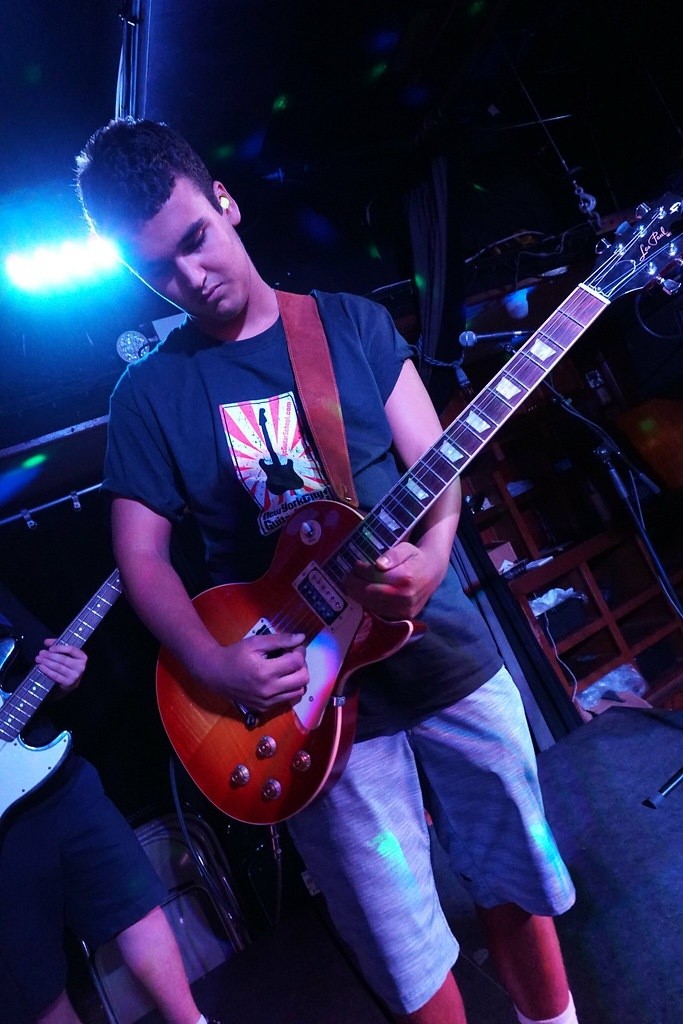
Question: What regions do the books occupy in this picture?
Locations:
[525,555,554,570]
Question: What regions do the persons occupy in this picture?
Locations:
[0,587,208,1023]
[76,116,579,1023]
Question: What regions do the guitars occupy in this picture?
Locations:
[153,192,682,827]
[1,564,123,819]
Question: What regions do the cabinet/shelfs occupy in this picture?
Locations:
[433,378,683,729]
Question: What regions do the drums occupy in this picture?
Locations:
[168,751,326,946]
